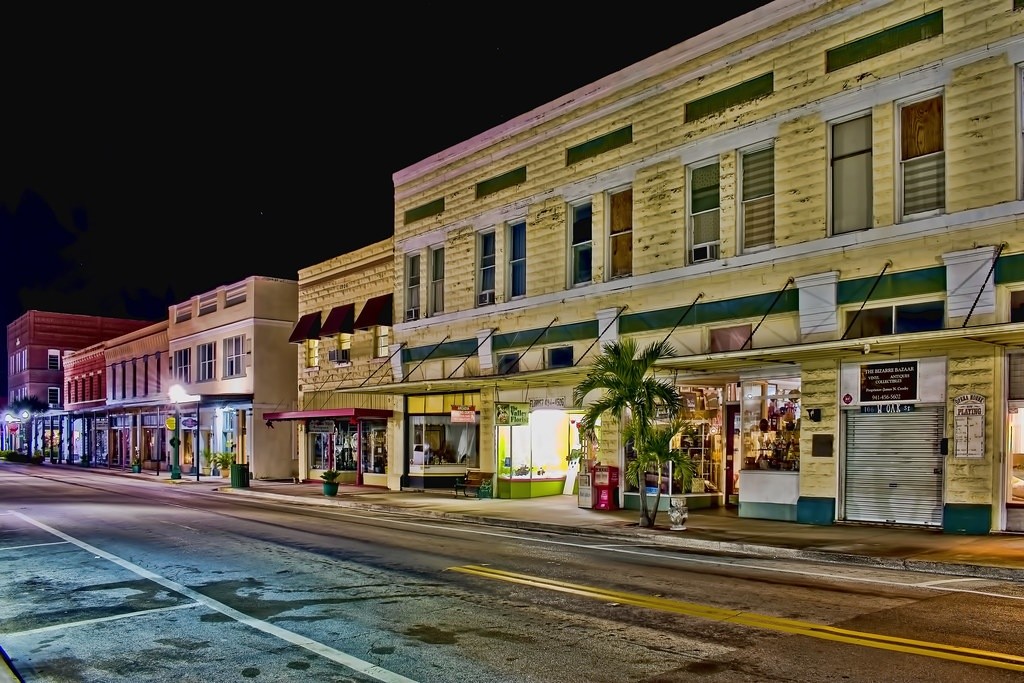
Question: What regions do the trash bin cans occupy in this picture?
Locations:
[231,464,249,487]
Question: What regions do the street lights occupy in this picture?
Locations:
[168,383,185,479]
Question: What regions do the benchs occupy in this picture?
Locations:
[454,471,494,497]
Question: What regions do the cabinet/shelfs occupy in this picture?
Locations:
[671,420,713,490]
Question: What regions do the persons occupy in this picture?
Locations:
[744,419,774,464]
[784,389,802,430]
[768,395,783,419]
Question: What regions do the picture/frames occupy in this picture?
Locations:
[679,386,698,408]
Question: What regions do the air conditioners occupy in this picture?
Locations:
[691,244,717,263]
[478,289,495,306]
[406,305,420,321]
[328,349,350,362]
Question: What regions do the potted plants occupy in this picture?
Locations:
[131,458,142,473]
[199,449,216,476]
[32,450,43,465]
[80,454,93,467]
[215,451,236,478]
[320,471,341,497]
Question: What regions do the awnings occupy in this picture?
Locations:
[354,294,393,331]
[320,303,355,339]
[288,311,321,345]
